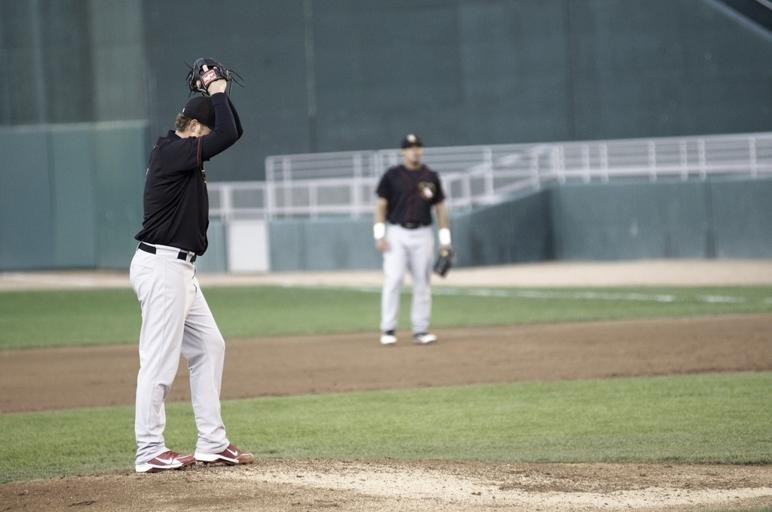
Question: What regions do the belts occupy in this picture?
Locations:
[139,243,196,262]
[390,222,430,228]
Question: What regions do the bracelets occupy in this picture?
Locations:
[373,224,386,240]
[437,228,452,246]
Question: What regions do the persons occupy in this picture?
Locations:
[128,58,257,473]
[373,133,453,345]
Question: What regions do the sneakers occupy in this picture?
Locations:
[379,331,397,344]
[193,438,255,465]
[134,448,197,474]
[412,333,438,344]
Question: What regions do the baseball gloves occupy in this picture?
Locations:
[433,249,454,275]
[188,56,231,93]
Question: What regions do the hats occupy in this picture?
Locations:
[401,134,423,148]
[180,97,217,130]
